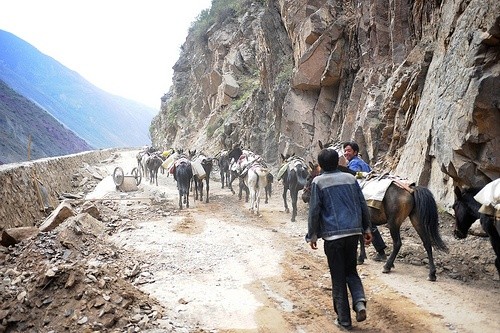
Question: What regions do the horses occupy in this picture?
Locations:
[137,146,174,185]
[442,161,500,250]
[302,161,440,281]
[282,154,308,221]
[175,148,212,209]
[218,140,272,216]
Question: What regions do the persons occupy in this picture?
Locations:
[343,142,388,261]
[305,149,372,330]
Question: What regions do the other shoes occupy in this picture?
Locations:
[371,252,387,260]
[335,319,351,327]
[355,302,367,322]
